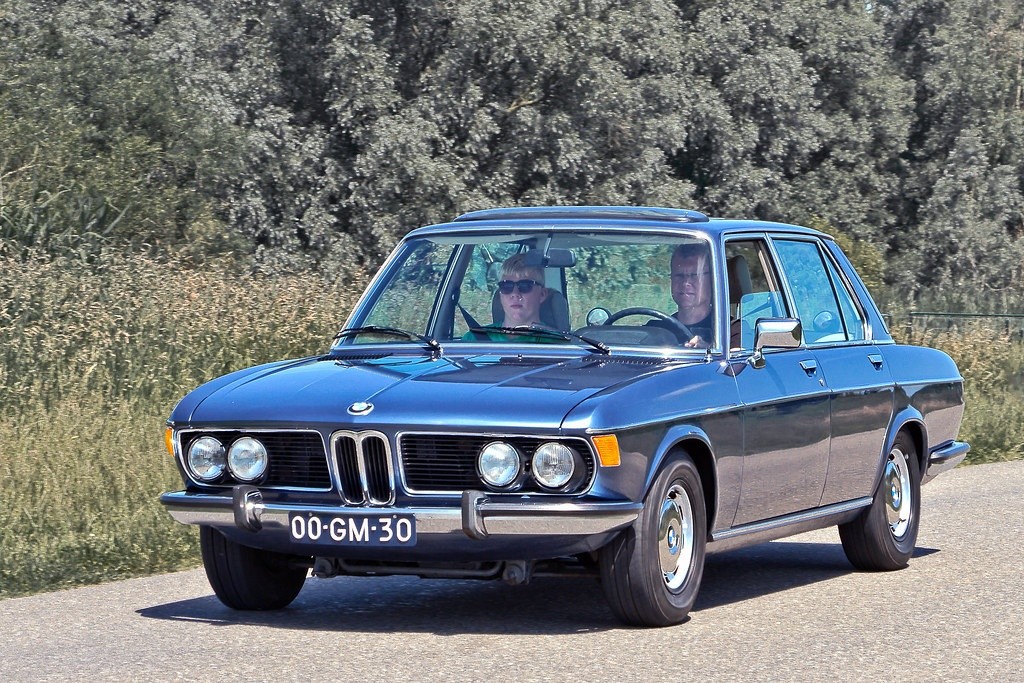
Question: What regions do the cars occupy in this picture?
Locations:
[158,206,972,625]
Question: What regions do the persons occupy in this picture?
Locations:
[462,253,567,345]
[642,244,751,352]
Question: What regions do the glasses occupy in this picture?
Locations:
[498,279,542,294]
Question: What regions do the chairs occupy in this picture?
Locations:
[723,255,754,319]
[491,286,571,335]
